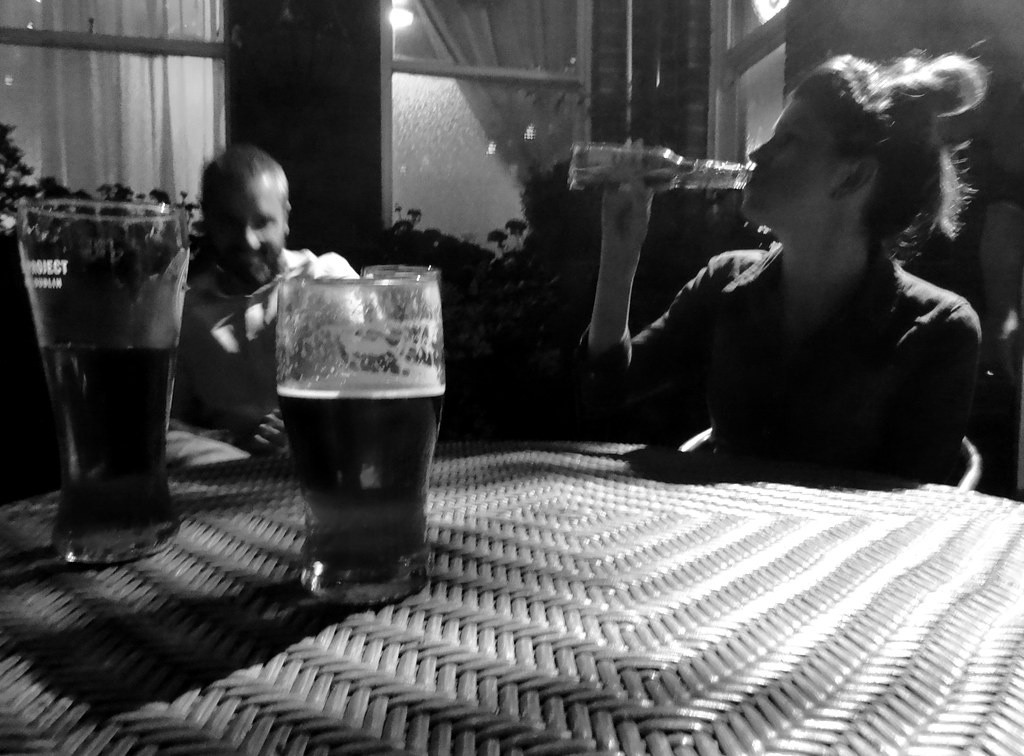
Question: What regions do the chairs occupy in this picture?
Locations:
[679,429,983,488]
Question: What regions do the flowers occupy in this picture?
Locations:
[485,219,526,258]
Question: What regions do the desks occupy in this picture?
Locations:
[1,443,1024,756]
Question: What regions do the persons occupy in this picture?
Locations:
[169,144,362,449]
[573,54,980,485]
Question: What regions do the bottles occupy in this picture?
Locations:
[567,141,753,193]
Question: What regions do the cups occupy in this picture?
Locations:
[16,200,190,562]
[273,264,445,605]
